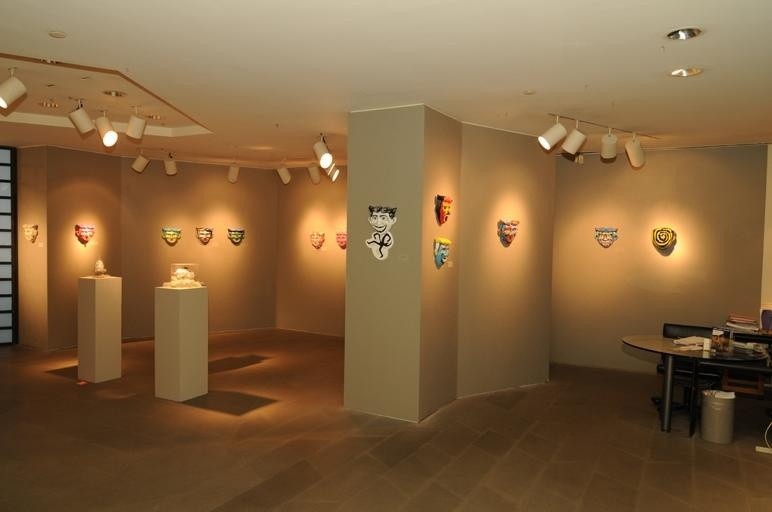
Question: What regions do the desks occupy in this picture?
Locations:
[622,334,772,431]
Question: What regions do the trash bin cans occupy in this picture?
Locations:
[702,390,735,443]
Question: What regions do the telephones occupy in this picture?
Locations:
[734,342,763,354]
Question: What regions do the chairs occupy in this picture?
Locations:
[652,320,713,420]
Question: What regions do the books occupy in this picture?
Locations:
[726,313,759,334]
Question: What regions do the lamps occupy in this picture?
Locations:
[228,162,239,184]
[277,133,340,185]
[0,67,27,110]
[68,98,177,176]
[538,113,658,168]
